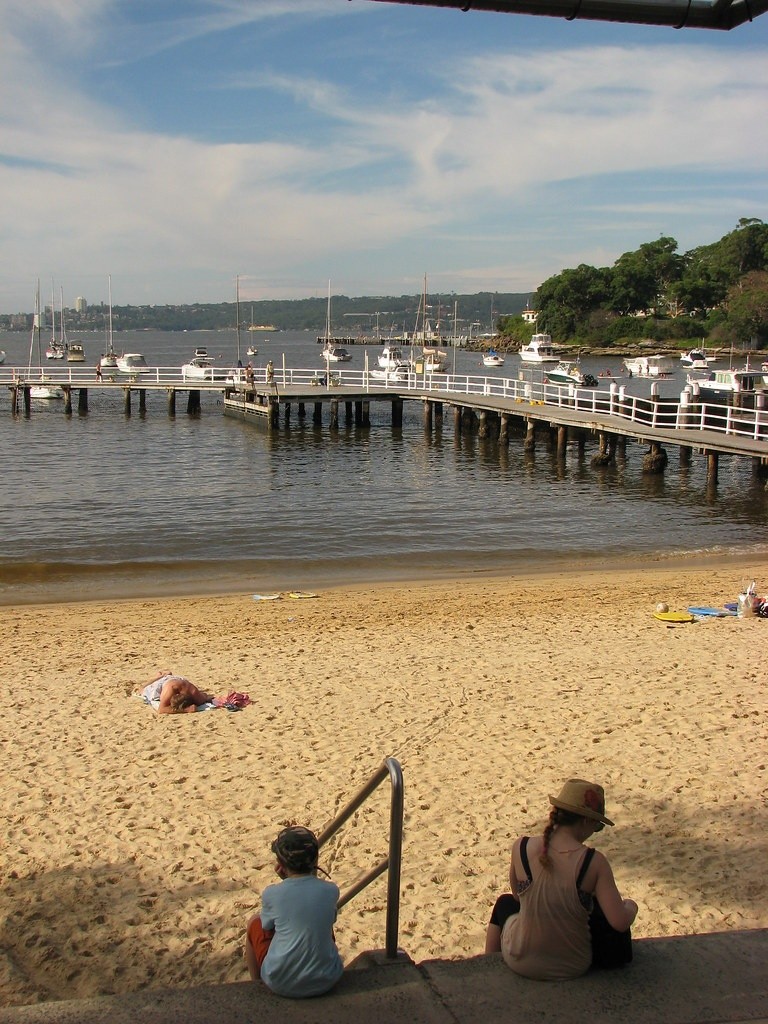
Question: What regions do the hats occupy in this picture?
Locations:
[548,779,615,826]
[271,826,320,873]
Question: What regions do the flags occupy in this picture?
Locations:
[489,345,498,356]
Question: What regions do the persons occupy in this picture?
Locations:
[95,362,103,383]
[265,360,274,385]
[629,365,667,379]
[320,367,333,385]
[245,826,343,997]
[243,364,255,387]
[139,670,212,714]
[485,779,638,982]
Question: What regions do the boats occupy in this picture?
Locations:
[249,324,279,331]
[680,348,717,368]
[686,366,768,403]
[544,360,599,386]
[370,367,416,383]
[181,357,226,379]
[377,346,411,367]
[116,353,150,372]
[624,357,675,374]
[518,334,560,362]
[194,346,208,356]
[66,338,86,361]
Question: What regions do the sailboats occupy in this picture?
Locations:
[24,277,65,399]
[226,273,256,382]
[46,276,70,358]
[100,273,118,367]
[320,278,352,362]
[483,293,504,366]
[247,305,258,355]
[409,270,450,371]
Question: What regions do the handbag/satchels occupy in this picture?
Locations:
[588,896,633,966]
[737,587,758,618]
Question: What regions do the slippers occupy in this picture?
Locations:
[223,702,239,712]
